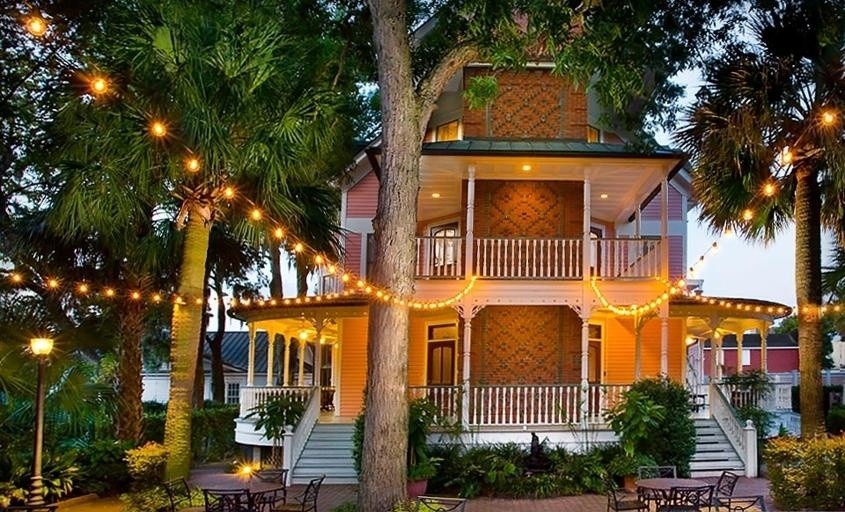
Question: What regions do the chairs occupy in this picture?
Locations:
[162,467,327,511]
[413,495,467,512]
[600,464,768,512]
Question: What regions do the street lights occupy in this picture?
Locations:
[26,335,56,506]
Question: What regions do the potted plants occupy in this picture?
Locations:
[606,452,662,492]
[405,394,445,497]
